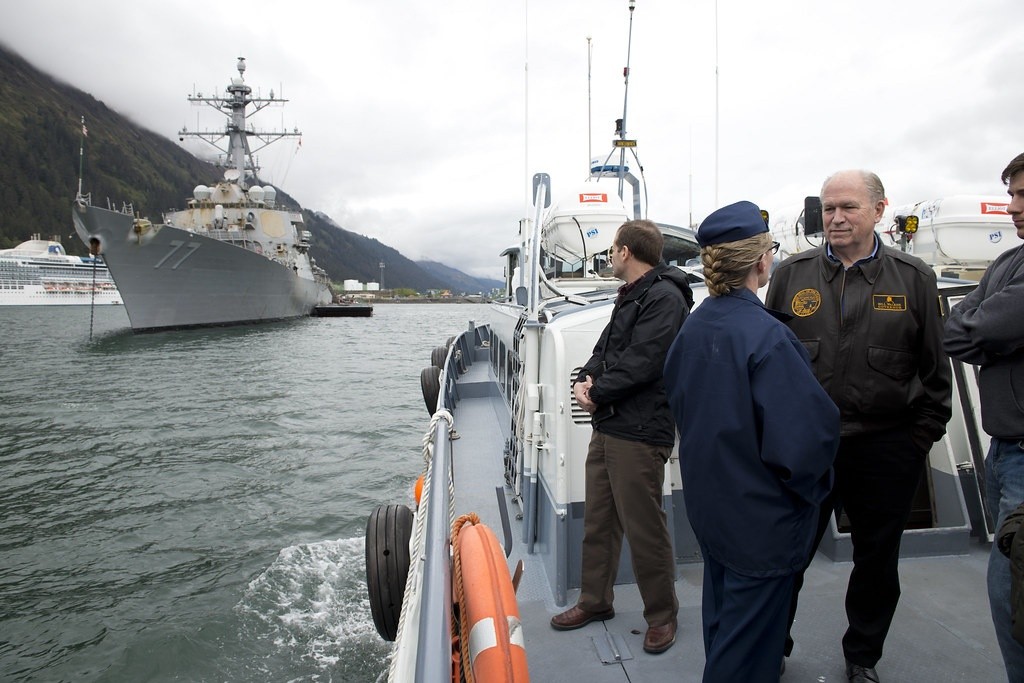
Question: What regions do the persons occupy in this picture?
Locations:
[551,219,694,654]
[765,170,953,683]
[666,201,839,683]
[945,153,1024,683]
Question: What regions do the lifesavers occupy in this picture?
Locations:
[450,524,530,683]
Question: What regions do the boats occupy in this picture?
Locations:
[312,299,374,318]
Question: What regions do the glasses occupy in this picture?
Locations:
[757,242,780,264]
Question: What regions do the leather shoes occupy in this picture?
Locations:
[550,604,615,630]
[643,618,678,652]
[846,660,879,683]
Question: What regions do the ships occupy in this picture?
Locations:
[70,56,334,337]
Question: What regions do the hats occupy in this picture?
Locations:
[695,201,769,248]
